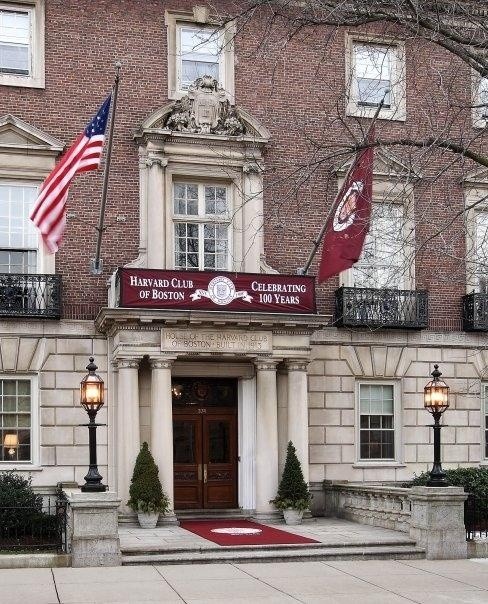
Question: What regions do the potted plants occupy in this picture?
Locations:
[269,439,314,525]
[125,441,172,529]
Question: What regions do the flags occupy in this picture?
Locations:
[30,93,112,252]
[317,126,377,284]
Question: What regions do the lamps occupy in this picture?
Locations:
[2,434,21,457]
[80,357,110,492]
[423,363,451,487]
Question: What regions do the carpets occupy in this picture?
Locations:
[178,520,323,546]
